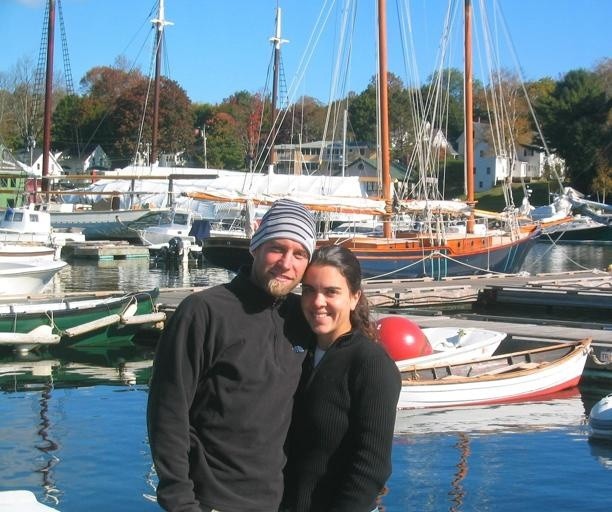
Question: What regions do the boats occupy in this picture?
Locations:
[395,327,593,420]
[0,288,159,346]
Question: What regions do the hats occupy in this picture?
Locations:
[249,199,317,264]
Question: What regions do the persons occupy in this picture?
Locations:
[279,243,403,511]
[146,198,316,512]
